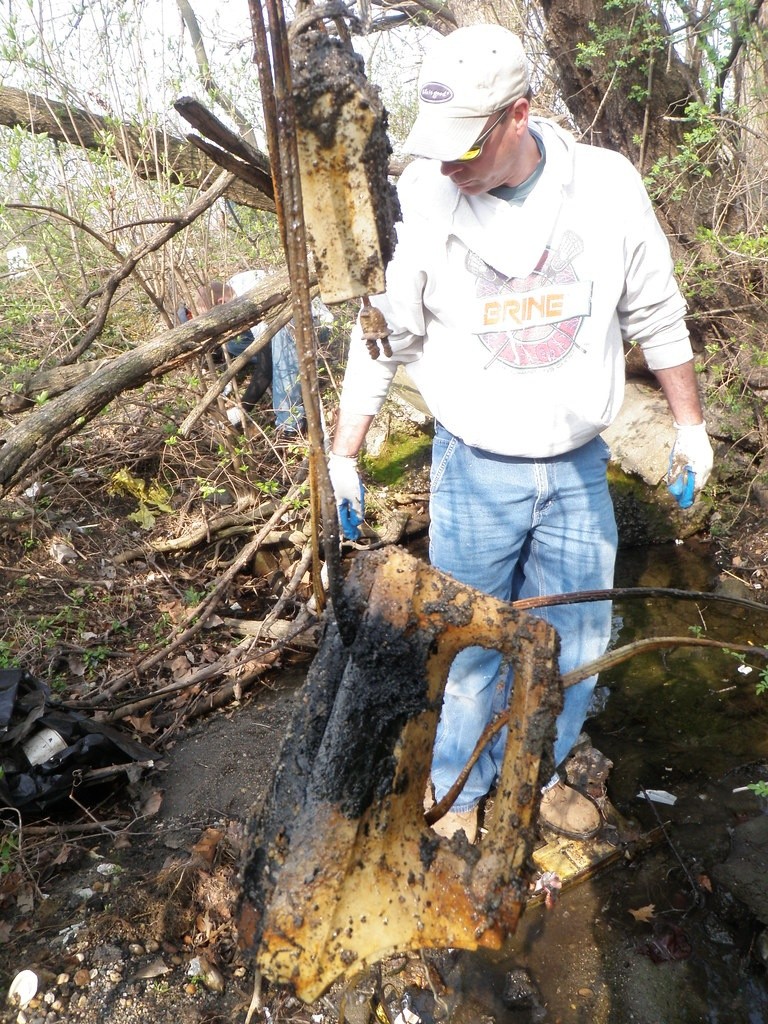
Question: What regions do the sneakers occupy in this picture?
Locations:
[537,780,601,840]
[424,782,477,847]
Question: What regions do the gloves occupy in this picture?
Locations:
[223,382,233,397]
[226,406,240,426]
[326,452,365,540]
[669,418,714,508]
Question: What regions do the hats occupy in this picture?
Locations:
[400,24,530,163]
[176,308,187,324]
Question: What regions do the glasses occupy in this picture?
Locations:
[453,107,512,165]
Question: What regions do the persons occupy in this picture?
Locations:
[196,270,334,455]
[327,24,714,845]
